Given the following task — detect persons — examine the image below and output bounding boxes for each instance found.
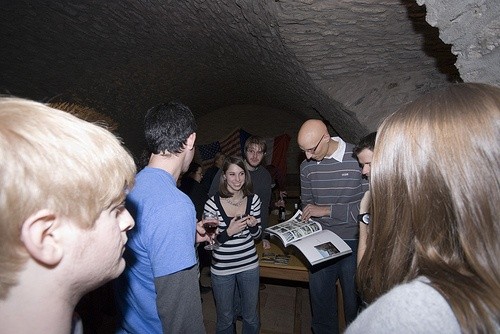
[202,156,262,334]
[0,96,137,334]
[180,119,377,334]
[341,83,500,334]
[115,103,219,333]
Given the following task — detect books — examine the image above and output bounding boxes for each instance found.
[263,209,352,266]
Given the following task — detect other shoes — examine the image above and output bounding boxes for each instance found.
[259,284,266,290]
[199,282,211,294]
[237,316,242,321]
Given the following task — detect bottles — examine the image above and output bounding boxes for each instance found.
[279,201,285,221]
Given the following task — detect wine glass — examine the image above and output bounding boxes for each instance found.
[202,211,219,250]
[235,214,247,238]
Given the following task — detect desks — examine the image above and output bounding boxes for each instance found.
[254,215,347,334]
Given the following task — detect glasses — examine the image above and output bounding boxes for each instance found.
[303,134,326,155]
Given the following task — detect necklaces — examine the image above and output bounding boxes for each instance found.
[224,196,246,207]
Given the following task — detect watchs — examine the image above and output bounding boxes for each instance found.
[358,212,370,225]
